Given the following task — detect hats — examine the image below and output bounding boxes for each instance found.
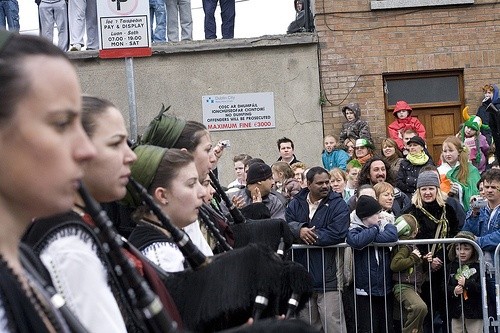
[407,136,425,147]
[247,162,273,183]
[447,231,479,263]
[141,103,186,147]
[355,196,382,219]
[417,171,440,188]
[351,139,375,149]
[461,106,490,164]
[394,217,411,237]
[116,144,167,210]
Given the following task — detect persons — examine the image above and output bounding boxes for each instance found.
[165,0,193,41]
[202,0,236,40]
[99,103,316,329]
[387,100,427,151]
[201,107,500,333]
[0,0,21,33]
[34,0,98,51]
[19,94,321,333]
[476,84,500,145]
[0,29,96,333]
[149,0,167,41]
[339,103,373,146]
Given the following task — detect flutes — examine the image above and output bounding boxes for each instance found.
[78,170,314,333]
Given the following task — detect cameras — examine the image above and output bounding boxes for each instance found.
[471,196,486,207]
[219,140,230,147]
